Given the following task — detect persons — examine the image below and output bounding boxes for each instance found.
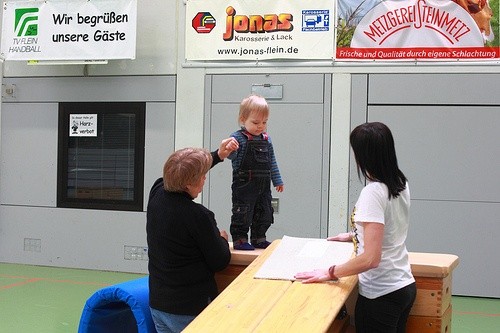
[226,96,284,250]
[145,137,239,333]
[295,122,417,333]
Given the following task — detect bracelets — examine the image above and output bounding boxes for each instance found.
[329,265,337,279]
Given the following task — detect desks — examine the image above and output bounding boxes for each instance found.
[217,243,460,333]
[180,236,356,333]
